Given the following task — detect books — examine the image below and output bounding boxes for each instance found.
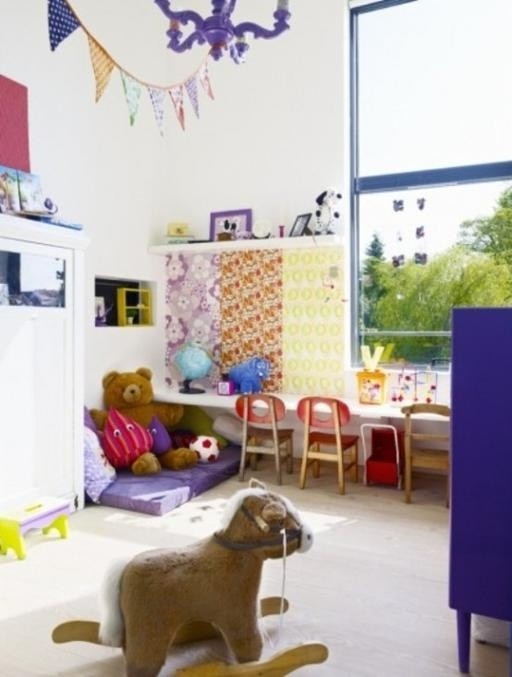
[17,169,51,214]
[37,198,84,230]
[0,164,21,215]
[19,207,48,217]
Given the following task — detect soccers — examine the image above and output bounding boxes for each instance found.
[189,435,219,463]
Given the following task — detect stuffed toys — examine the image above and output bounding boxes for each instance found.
[228,357,270,395]
[88,367,198,476]
[314,188,342,235]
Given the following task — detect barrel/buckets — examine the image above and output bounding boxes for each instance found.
[356,368,388,405]
